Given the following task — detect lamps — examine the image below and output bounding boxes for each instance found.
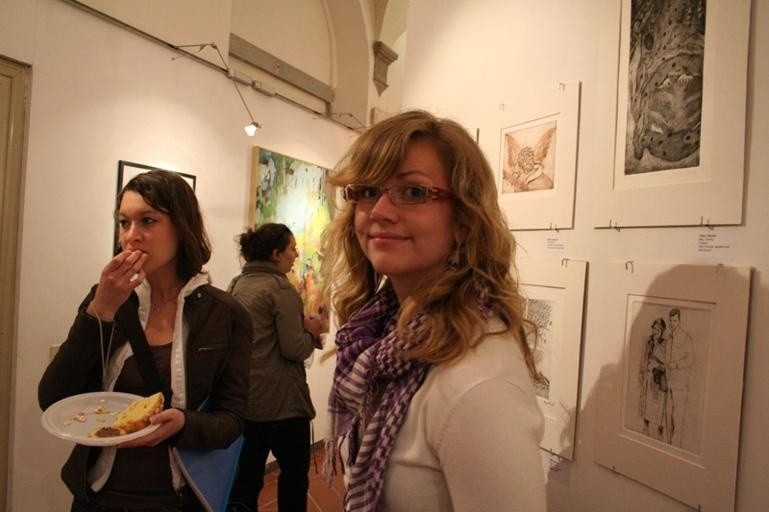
[166,41,264,140]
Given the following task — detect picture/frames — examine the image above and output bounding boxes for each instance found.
[482,79,585,233]
[508,255,589,465]
[112,159,198,257]
[589,0,756,232]
[591,255,759,512]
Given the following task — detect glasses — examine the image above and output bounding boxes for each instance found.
[341,181,450,205]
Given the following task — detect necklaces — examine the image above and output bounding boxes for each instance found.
[149,286,180,313]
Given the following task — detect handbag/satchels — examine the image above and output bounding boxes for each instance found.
[179,448,264,511]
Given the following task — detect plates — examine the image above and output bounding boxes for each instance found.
[41,390,161,450]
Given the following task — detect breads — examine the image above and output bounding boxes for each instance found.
[93,390,164,439]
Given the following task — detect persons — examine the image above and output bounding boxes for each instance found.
[318,107,550,511]
[36,168,254,511]
[637,317,672,442]
[226,222,321,512]
[665,306,694,448]
